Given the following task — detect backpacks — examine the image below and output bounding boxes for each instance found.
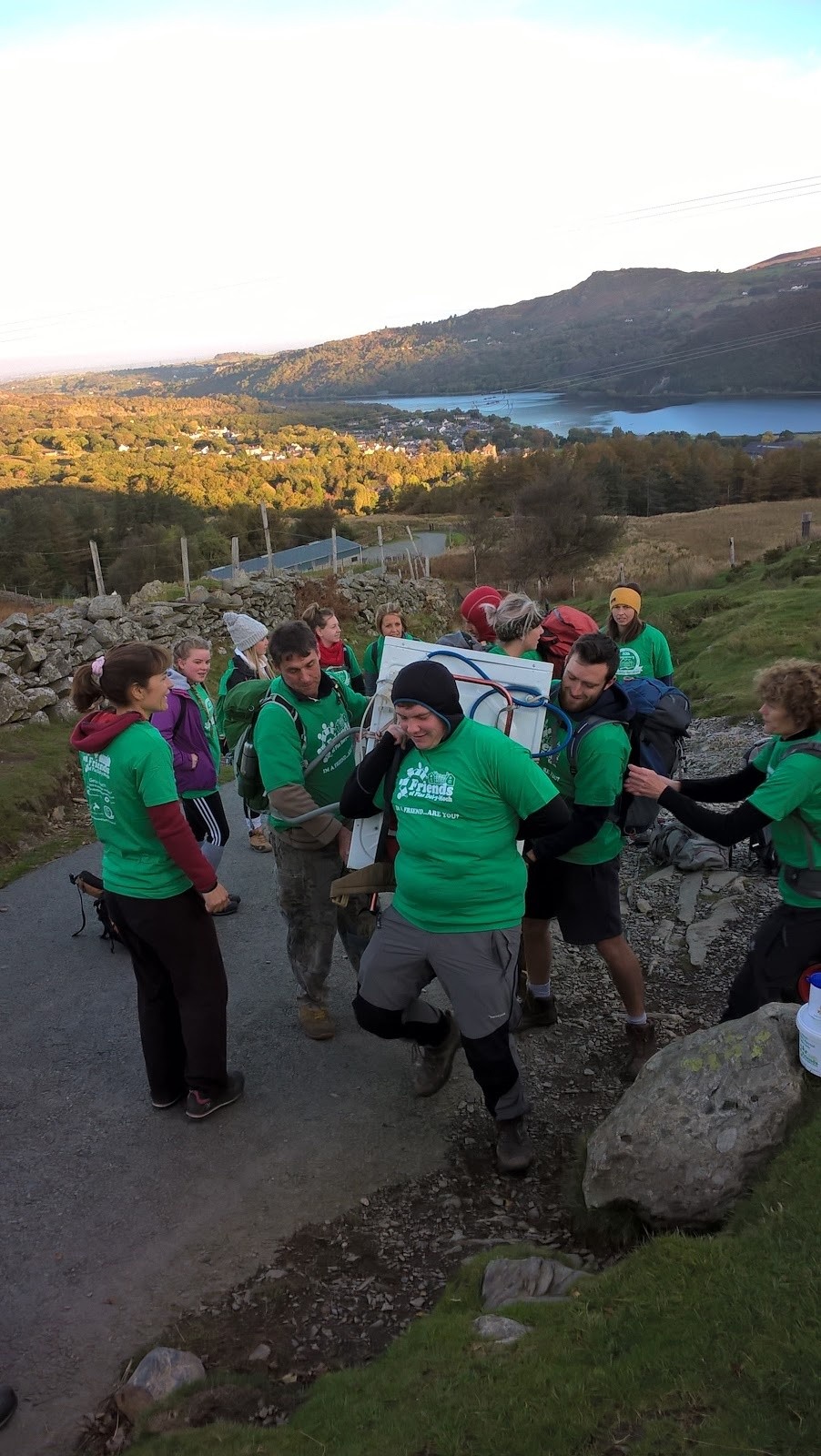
[537,605,599,681]
[650,821,728,873]
[221,679,269,811]
[568,676,692,835]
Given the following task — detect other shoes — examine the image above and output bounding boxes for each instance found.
[186,1070,246,1119]
[152,1094,179,1107]
[212,895,241,916]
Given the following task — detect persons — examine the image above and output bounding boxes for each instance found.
[66,643,247,1118]
[621,658,821,1023]
[214,583,673,853]
[339,658,572,1173]
[509,631,658,1081]
[150,635,241,916]
[252,622,382,1039]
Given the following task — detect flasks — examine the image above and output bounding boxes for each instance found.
[240,739,258,780]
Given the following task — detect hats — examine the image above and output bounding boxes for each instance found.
[223,612,268,652]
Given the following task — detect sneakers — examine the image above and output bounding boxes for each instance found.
[624,1019,656,1080]
[411,1009,464,1097]
[517,988,558,1032]
[248,827,272,852]
[495,1121,531,1179]
[296,984,334,1040]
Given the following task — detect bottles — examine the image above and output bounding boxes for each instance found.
[795,972,821,1077]
